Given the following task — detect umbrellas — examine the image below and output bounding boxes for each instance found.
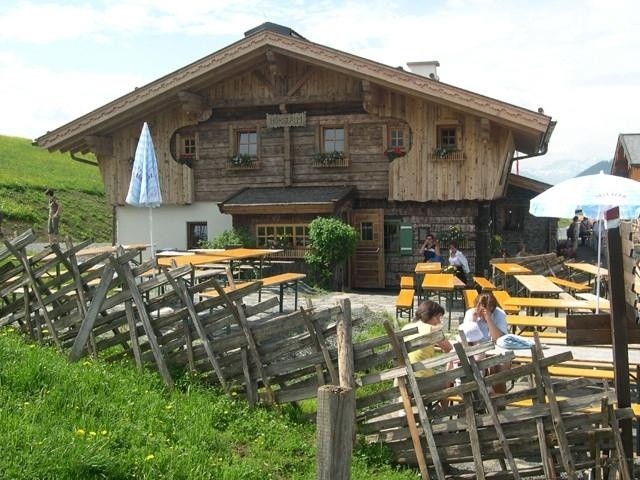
[529,169,640,314]
[125,120,163,278]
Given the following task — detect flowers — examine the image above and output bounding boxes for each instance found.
[173,144,456,169]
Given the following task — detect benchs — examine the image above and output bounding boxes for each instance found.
[139,245,307,318]
[385,261,640,421]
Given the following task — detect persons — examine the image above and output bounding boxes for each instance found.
[447,240,471,285]
[44,189,61,248]
[393,300,454,417]
[420,233,446,265]
[565,215,592,255]
[458,293,514,410]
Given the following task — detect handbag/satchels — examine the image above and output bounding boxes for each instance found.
[495,333,533,351]
